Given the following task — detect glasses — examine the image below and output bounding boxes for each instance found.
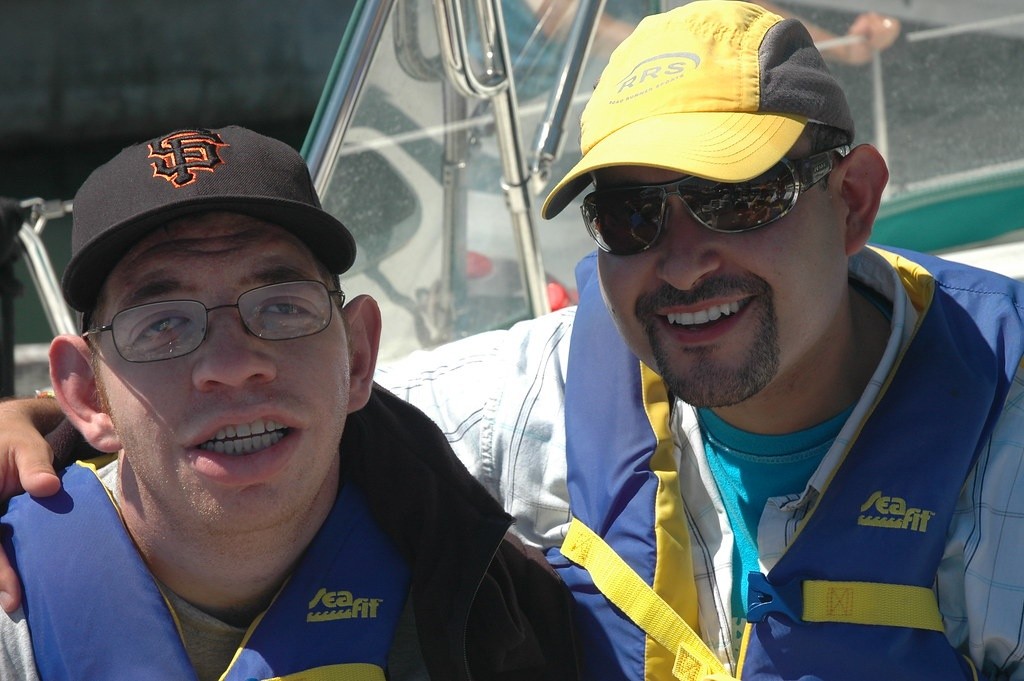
[580,139,848,257]
[84,278,348,365]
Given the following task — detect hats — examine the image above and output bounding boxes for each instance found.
[541,2,854,221]
[62,124,357,314]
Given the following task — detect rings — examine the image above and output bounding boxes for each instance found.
[882,19,892,30]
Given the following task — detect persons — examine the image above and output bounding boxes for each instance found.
[496,1,902,319]
[4,0,1024,680]
[0,123,622,681]
